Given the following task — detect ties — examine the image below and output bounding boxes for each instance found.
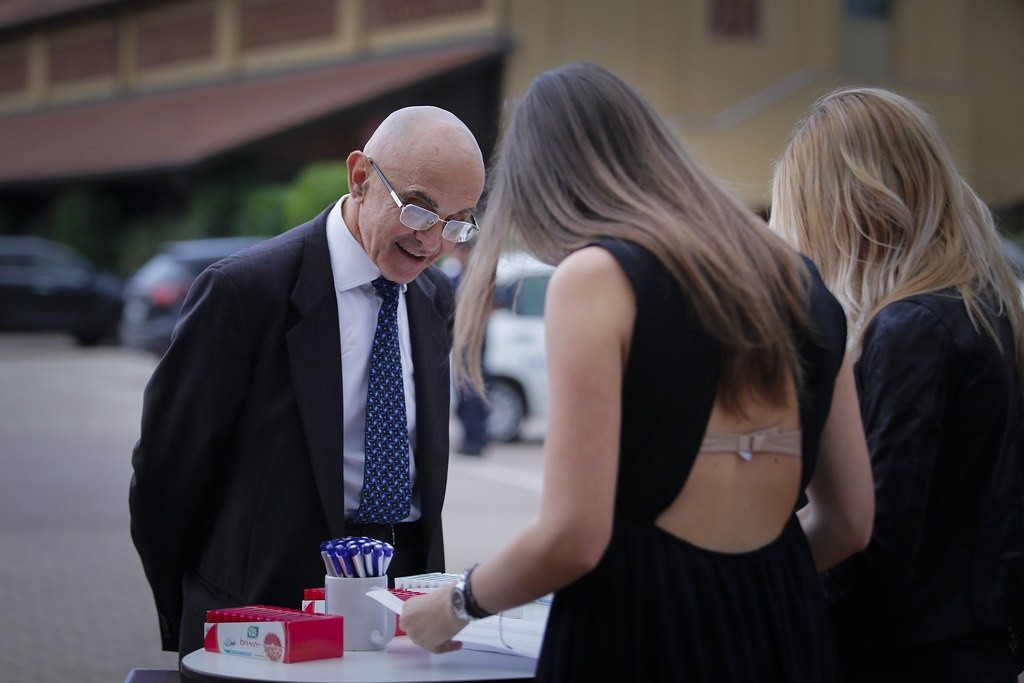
[353,275,413,524]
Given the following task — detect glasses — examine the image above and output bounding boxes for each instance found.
[366,156,480,243]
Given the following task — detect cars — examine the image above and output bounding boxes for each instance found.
[0,232,130,348]
[119,235,277,357]
[432,248,560,450]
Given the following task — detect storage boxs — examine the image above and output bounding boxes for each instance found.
[302,599,406,637]
[203,615,344,663]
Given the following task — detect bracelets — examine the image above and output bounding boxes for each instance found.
[463,565,492,619]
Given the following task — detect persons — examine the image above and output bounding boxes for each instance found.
[399,62,877,683]
[766,85,1023,683]
[128,106,486,683]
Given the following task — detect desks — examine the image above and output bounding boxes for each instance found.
[179,632,538,683]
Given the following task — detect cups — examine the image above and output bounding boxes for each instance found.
[325,574,397,651]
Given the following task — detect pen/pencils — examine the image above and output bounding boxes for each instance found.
[320,536,393,578]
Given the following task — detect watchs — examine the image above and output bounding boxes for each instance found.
[451,564,477,621]
